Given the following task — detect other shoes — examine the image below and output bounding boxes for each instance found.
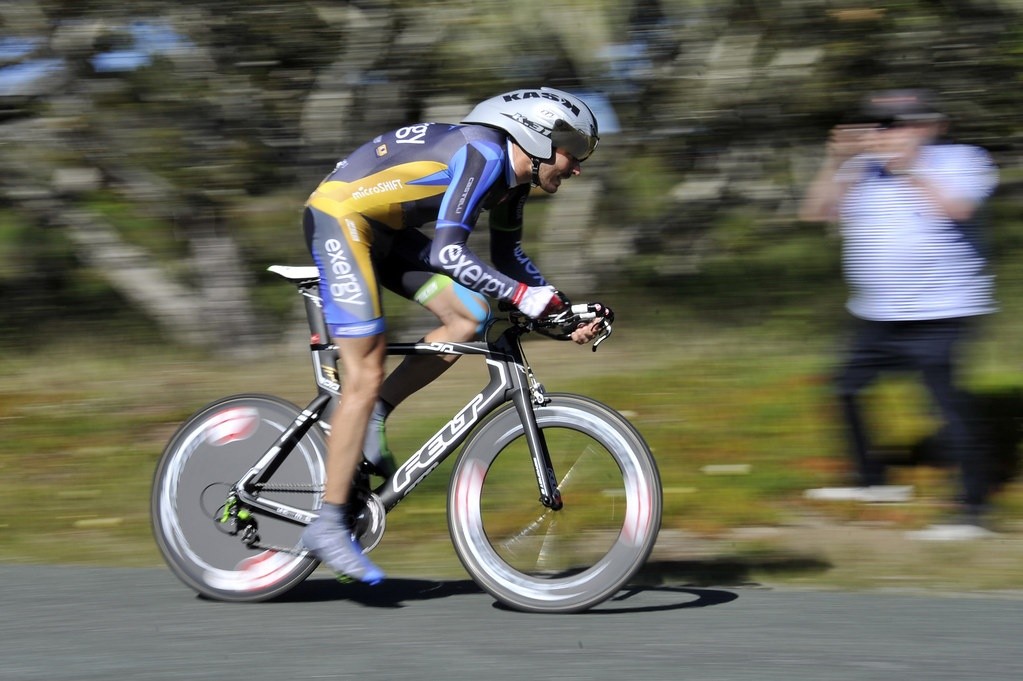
[360,404,397,479]
[303,502,384,585]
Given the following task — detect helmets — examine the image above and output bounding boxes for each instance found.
[459,86,600,162]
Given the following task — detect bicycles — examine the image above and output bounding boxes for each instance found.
[150,265,662,614]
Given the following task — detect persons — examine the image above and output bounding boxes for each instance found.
[795,84,1004,540]
[301,86,604,586]
